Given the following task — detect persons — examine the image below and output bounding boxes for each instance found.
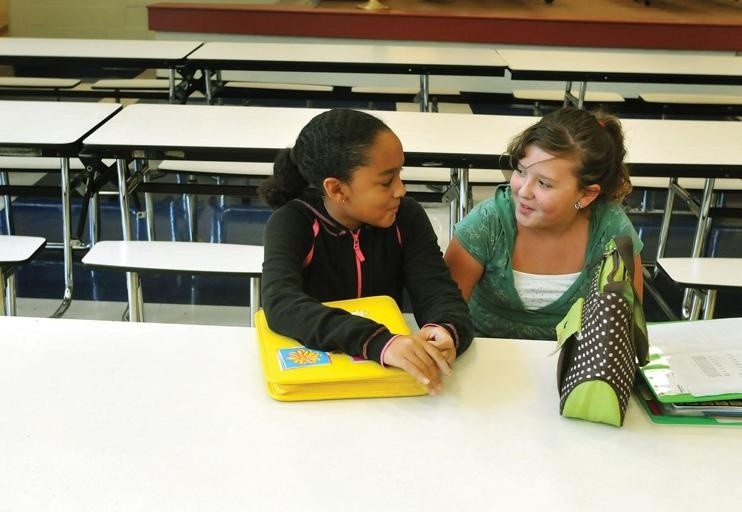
[254,105,477,399]
[442,104,647,341]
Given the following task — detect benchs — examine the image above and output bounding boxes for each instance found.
[0,37,742,328]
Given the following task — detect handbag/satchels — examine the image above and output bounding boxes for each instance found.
[551,234,650,428]
[255,295,431,402]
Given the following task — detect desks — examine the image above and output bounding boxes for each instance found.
[0,315,742,440]
